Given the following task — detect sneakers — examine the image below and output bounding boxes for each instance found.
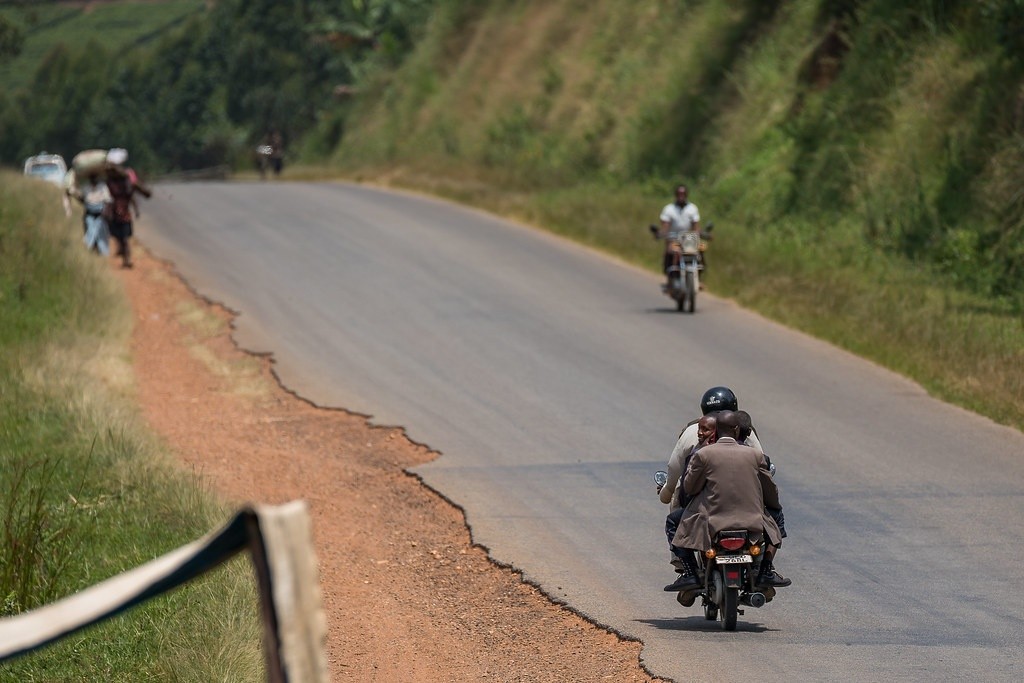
[663,574,700,591]
[755,570,792,587]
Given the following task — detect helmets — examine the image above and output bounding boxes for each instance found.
[701,387,739,416]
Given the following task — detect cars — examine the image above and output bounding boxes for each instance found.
[24,154,69,190]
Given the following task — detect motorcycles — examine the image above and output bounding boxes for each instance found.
[652,464,776,633]
[649,220,717,316]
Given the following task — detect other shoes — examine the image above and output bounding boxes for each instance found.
[756,586,775,602]
[676,591,695,607]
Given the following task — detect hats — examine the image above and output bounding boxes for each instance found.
[107,147,128,165]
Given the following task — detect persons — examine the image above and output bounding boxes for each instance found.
[658,387,791,593]
[253,129,286,179]
[652,185,713,288]
[67,157,153,270]
